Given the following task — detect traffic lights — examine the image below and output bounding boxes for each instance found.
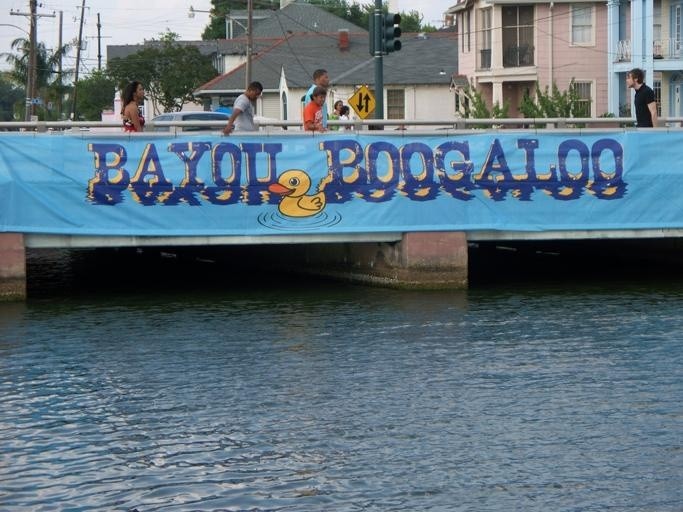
[379,11,401,54]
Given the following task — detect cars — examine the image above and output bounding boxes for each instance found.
[143,111,236,132]
[47,117,92,131]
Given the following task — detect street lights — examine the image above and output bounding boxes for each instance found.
[187,0,252,97]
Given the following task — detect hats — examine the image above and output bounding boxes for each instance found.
[310,87,326,100]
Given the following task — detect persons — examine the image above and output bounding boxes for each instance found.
[332,100,349,131]
[224,82,263,136]
[626,69,658,128]
[122,81,144,132]
[395,125,407,130]
[304,69,329,131]
